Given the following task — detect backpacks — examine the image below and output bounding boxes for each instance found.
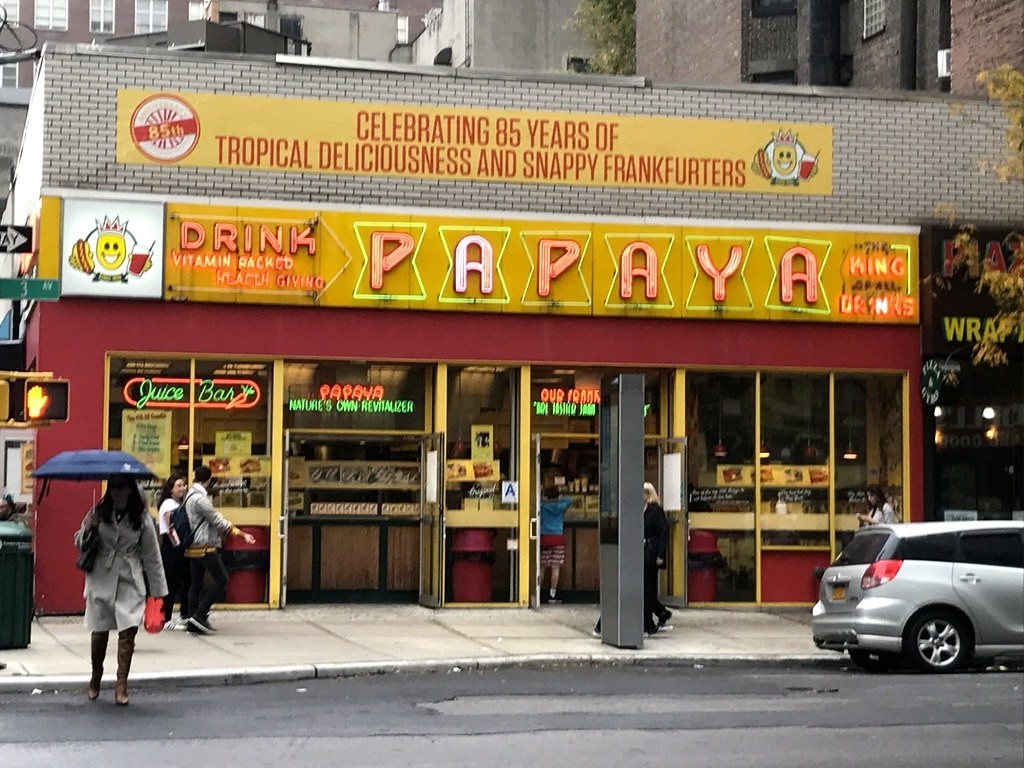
[164,493,208,549]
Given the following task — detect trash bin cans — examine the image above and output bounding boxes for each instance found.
[226,526,267,602]
[687,530,720,602]
[450,529,494,604]
[0,521,33,649]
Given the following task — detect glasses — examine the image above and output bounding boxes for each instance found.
[108,480,128,491]
[174,485,187,490]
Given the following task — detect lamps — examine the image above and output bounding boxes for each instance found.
[843,375,858,460]
[760,380,770,459]
[804,373,817,457]
[714,373,727,456]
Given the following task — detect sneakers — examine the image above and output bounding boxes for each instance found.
[163,619,187,630]
[186,623,202,635]
[189,612,217,634]
[177,616,189,625]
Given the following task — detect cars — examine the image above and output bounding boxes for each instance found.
[810,520,1024,672]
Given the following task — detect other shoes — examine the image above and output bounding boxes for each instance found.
[547,593,562,604]
[592,630,601,639]
[649,626,673,637]
[657,611,671,625]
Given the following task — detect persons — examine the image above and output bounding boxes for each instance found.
[157,473,187,631]
[591,591,674,638]
[539,486,572,604]
[73,475,169,706]
[643,481,672,633]
[29,448,160,513]
[178,466,256,635]
[856,483,896,527]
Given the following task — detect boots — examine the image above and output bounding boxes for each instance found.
[88,637,106,699]
[116,638,134,704]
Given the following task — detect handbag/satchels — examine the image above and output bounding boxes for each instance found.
[75,505,100,572]
[145,595,165,633]
